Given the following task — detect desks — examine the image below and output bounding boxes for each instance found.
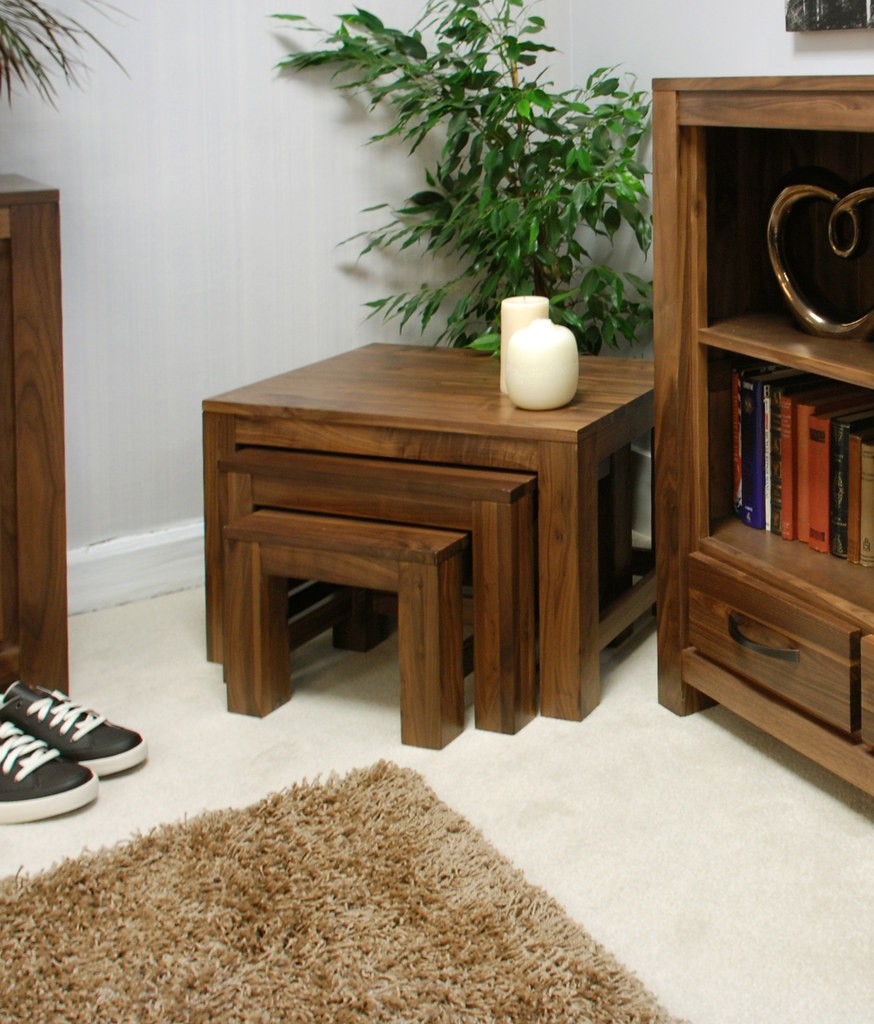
[652,76,874,796]
[0,173,69,699]
[202,341,656,723]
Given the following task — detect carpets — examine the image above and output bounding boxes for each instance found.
[0,757,698,1024]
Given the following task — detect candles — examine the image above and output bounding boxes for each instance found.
[500,295,549,394]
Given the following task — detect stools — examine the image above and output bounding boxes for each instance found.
[216,445,634,735]
[221,510,471,752]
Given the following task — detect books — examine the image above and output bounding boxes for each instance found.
[730,365,874,572]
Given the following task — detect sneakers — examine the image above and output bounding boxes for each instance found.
[0,678,147,777]
[0,718,102,825]
